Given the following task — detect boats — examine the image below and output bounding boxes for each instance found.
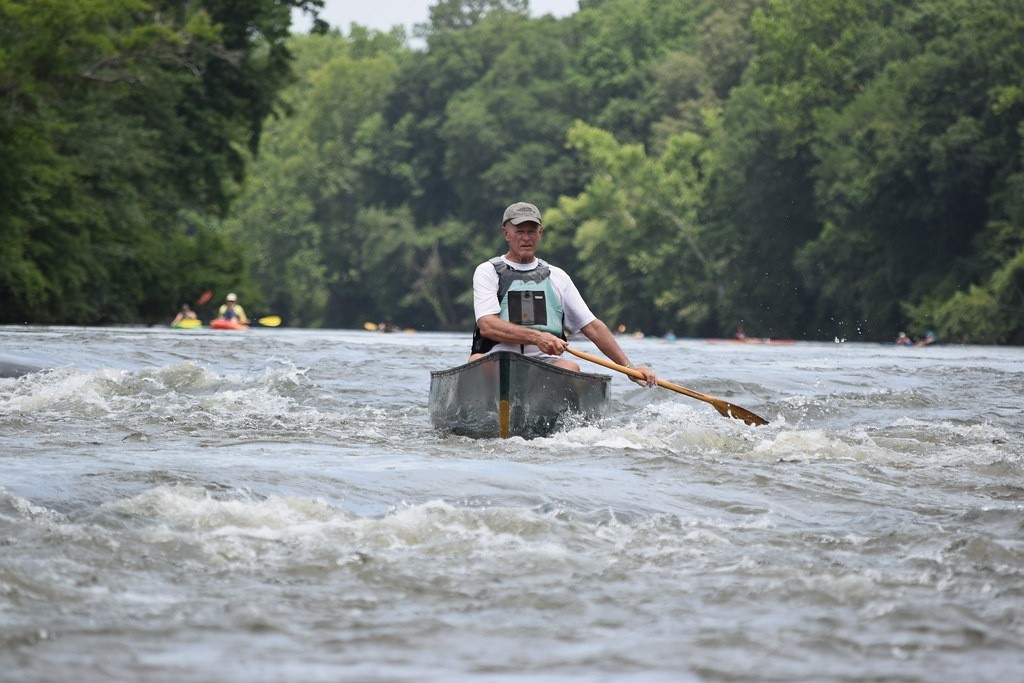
[210,318,246,330]
[708,338,798,345]
[175,319,201,329]
[898,332,937,347]
[428,350,612,439]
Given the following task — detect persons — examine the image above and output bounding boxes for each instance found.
[736,326,747,339]
[468,202,658,388]
[218,293,247,325]
[175,305,197,320]
[896,332,912,345]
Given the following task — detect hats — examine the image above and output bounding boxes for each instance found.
[227,294,236,301]
[502,202,542,225]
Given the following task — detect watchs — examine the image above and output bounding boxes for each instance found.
[625,361,634,367]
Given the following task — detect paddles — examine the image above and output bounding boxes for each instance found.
[177,315,282,329]
[562,344,772,429]
[364,321,415,334]
[180,290,213,321]
